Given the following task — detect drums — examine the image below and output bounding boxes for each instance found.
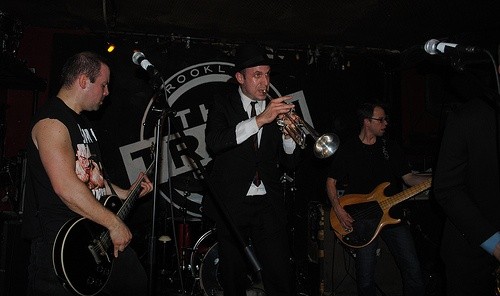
[166,217,202,251]
[190,228,256,296]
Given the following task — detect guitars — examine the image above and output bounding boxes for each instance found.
[329,178,433,249]
[52,140,160,296]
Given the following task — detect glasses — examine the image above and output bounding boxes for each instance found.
[365,114,390,124]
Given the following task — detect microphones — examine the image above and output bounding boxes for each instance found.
[131,51,160,77]
[423,39,480,55]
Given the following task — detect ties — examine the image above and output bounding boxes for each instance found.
[250,100,261,187]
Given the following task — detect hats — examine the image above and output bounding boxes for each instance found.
[225,44,276,75]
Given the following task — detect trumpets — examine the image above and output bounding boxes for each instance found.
[263,89,339,160]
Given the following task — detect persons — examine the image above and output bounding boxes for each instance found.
[428,58,500,296]
[326,103,431,296]
[26,51,153,296]
[204,46,300,296]
